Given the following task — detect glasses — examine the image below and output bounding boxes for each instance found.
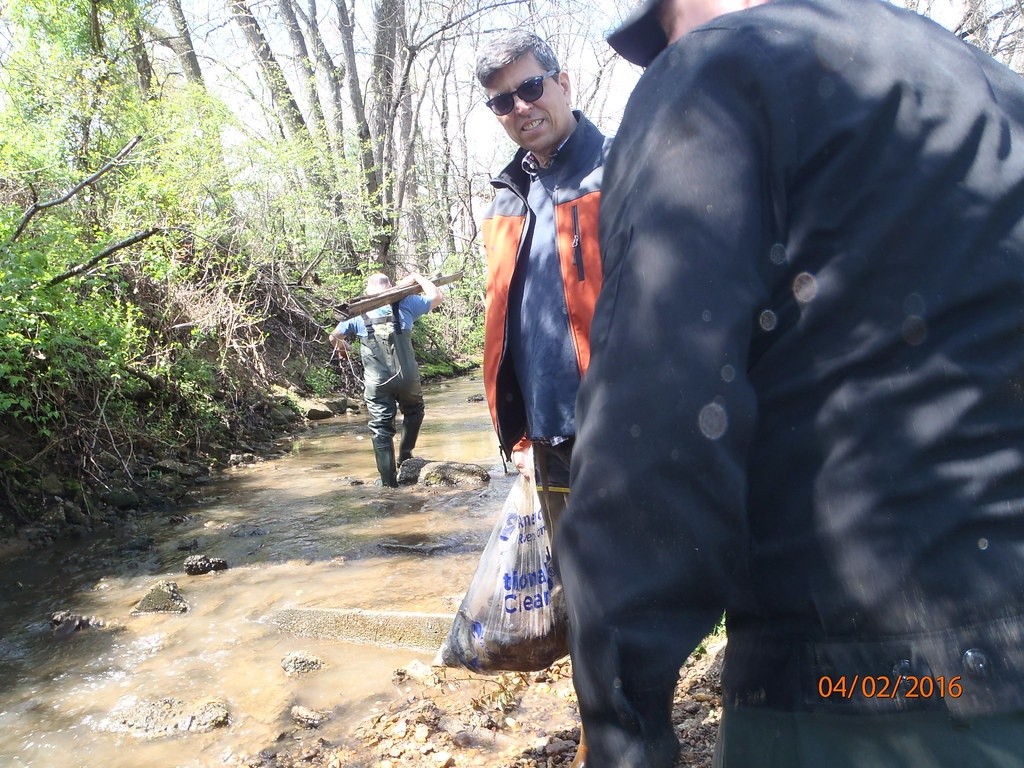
[485,68,557,116]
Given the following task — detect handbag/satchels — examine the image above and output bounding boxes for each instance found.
[441,451,572,673]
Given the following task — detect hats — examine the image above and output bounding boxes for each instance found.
[363,274,393,295]
[606,0,667,66]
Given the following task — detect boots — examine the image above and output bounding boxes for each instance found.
[373,436,397,489]
[396,412,423,469]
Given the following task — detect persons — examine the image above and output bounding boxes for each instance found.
[330,272,444,486]
[474,30,615,768]
[554,0,1024,768]
